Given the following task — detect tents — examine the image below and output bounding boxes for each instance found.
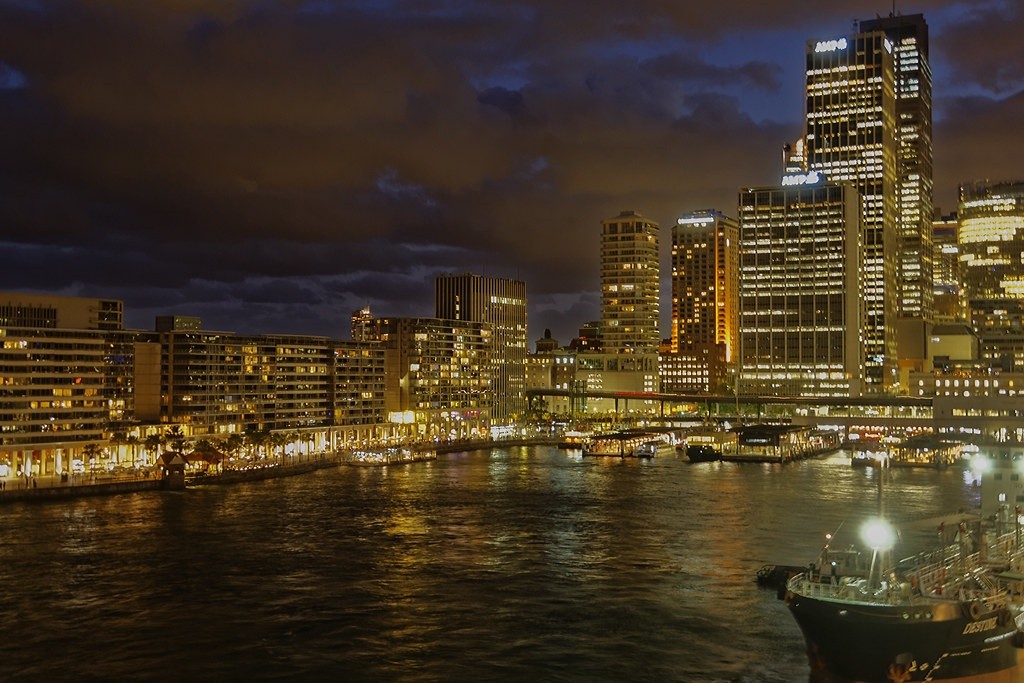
[184,447,230,469]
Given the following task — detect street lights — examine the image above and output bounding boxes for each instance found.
[855,449,995,581]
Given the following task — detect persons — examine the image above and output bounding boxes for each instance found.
[33,478,36,488]
[0,480,6,490]
